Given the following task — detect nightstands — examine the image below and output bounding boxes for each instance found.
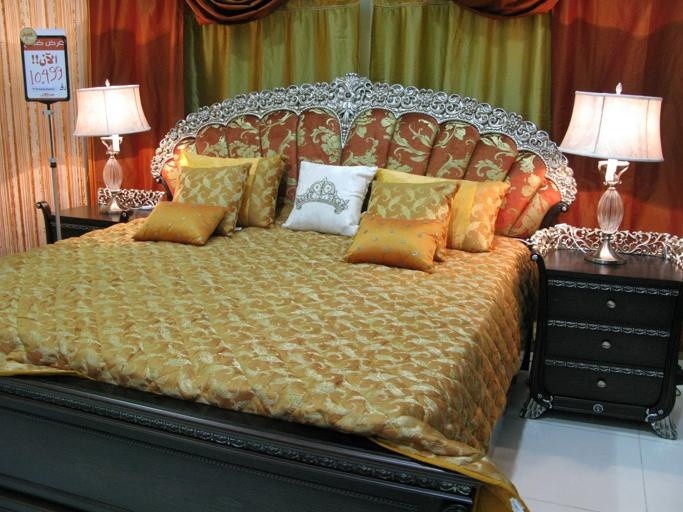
[518,247,682,441]
[34,201,151,245]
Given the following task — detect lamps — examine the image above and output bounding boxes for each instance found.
[71,77,151,215]
[555,83,664,266]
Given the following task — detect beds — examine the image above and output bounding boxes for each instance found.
[0,73,578,510]
